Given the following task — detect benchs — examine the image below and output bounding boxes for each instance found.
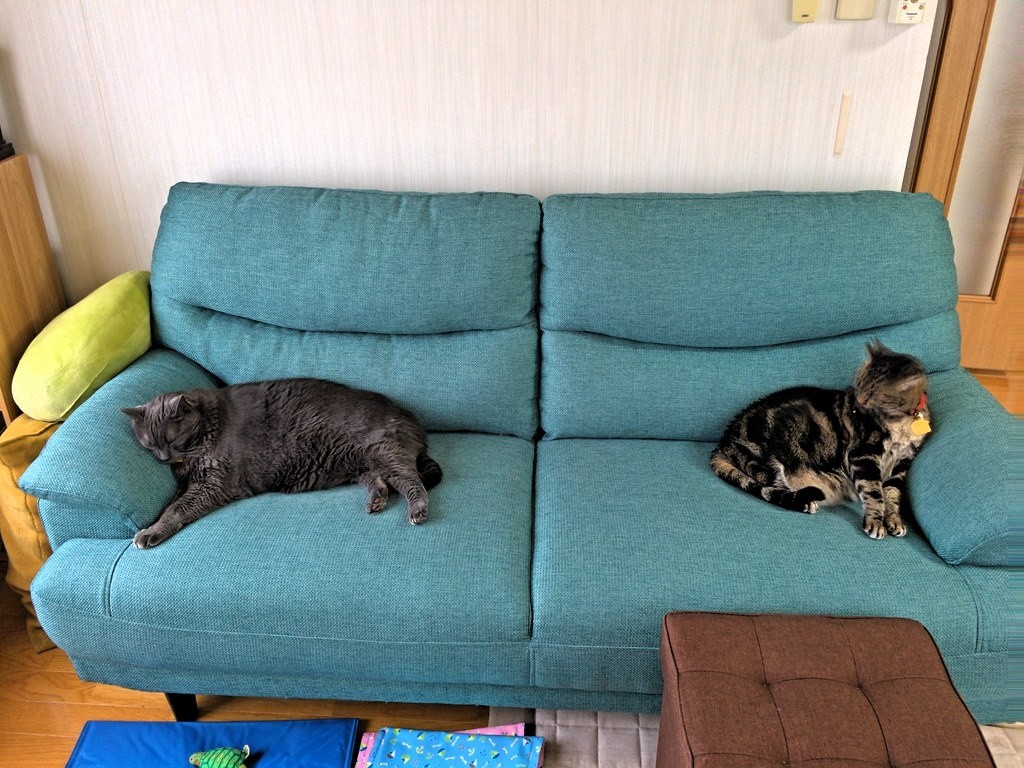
[654,613,999,768]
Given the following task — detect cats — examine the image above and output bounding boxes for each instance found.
[709,334,933,540]
[120,376,444,552]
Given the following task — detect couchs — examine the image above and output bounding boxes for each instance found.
[15,181,1024,722]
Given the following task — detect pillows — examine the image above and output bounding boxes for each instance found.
[12,269,152,421]
[0,413,62,655]
